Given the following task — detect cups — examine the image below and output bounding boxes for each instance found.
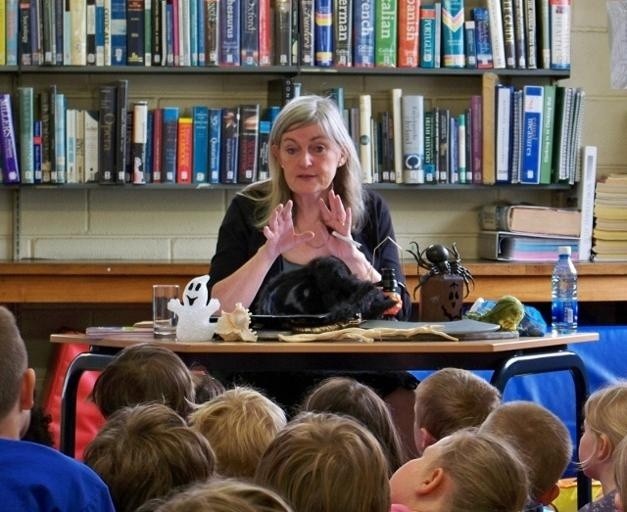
[417,273,463,323]
[151,283,178,338]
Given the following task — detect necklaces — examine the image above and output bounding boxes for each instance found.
[294,215,332,250]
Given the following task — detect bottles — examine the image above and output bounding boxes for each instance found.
[550,245,579,335]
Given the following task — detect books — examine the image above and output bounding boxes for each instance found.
[477,169,626,262]
[0,1,572,70]
[0,73,586,185]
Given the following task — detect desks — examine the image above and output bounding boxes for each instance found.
[52,328,600,512]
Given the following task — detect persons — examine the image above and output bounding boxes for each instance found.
[203,93,412,320]
[81,343,624,512]
[0,306,115,512]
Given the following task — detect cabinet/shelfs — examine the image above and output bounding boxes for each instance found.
[0,0,575,191]
